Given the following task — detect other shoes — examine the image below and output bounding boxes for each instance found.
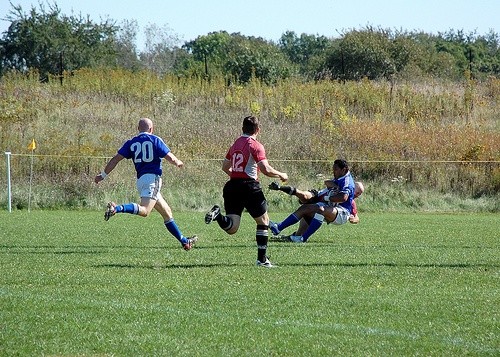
[281,231,297,242]
[205,205,221,224]
[289,235,309,243]
[269,220,281,236]
[257,255,279,268]
[269,182,280,190]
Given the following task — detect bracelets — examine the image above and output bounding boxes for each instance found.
[100,171,108,179]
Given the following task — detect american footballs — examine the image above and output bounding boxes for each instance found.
[327,187,341,207]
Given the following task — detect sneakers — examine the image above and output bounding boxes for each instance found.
[182,235,198,251]
[104,201,118,221]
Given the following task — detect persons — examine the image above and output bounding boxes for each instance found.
[95,118,198,251]
[268,159,355,243]
[269,181,364,240]
[204,116,288,268]
